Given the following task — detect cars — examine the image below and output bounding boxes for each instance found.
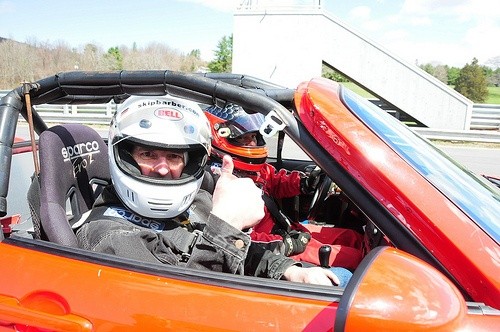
[0,68,499,332]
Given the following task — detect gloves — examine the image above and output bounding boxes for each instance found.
[282,230,311,257]
[300,169,326,194]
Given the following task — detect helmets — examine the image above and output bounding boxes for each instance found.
[108,92,210,218]
[203,103,269,175]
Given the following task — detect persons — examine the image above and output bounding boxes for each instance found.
[75,92,354,288]
[204,101,366,270]
